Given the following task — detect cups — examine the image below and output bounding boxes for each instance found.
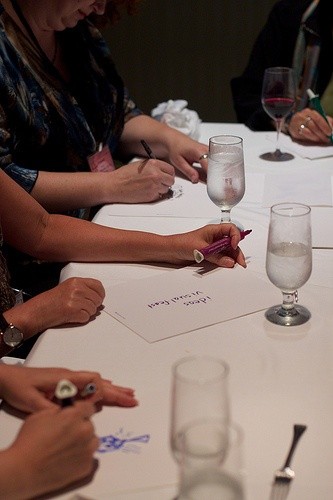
[170,358,230,469]
[167,420,247,500]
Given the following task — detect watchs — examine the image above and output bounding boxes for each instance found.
[0,312,25,349]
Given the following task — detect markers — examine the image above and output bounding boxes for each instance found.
[193,230,252,263]
[55,379,78,406]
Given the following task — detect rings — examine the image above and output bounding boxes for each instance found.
[297,124,306,135]
[305,117,311,125]
[199,154,207,160]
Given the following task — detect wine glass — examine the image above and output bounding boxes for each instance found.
[263,203,313,327]
[260,67,298,162]
[206,134,246,232]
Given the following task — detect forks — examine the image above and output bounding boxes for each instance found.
[269,422,308,500]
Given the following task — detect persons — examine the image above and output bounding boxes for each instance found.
[0,0,220,301]
[0,362,139,500]
[228,0,333,143]
[0,167,246,351]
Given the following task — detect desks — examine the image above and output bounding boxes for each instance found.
[0,123,333,500]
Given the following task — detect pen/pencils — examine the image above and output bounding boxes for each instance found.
[306,88,333,144]
[81,383,96,397]
[141,140,156,159]
